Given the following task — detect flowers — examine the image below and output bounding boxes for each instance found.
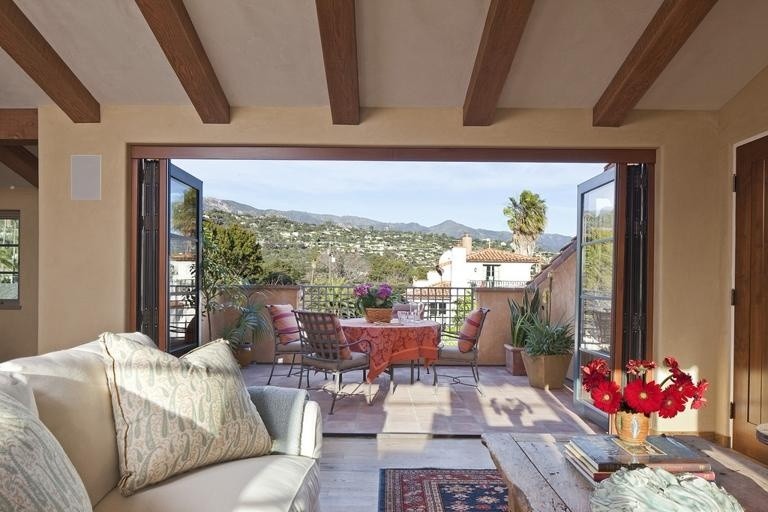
[580,357,709,418]
[351,283,400,315]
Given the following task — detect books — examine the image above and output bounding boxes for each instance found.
[563,433,717,488]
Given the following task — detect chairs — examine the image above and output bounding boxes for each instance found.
[265,304,327,388]
[388,302,430,382]
[292,309,373,414]
[433,306,490,387]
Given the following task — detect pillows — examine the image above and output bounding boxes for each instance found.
[97,332,273,497]
[458,307,483,352]
[269,304,301,344]
[298,311,353,359]
[0,368,96,512]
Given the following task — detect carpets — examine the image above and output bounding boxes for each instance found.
[378,468,513,512]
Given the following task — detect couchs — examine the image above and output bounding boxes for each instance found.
[0,331,323,512]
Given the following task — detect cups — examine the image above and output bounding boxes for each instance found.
[396,311,416,325]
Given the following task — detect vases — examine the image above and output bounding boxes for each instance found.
[615,410,650,445]
[364,308,393,323]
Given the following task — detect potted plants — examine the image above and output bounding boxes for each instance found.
[219,284,278,367]
[504,286,541,375]
[519,308,575,390]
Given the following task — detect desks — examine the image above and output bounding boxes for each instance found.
[477,433,768,512]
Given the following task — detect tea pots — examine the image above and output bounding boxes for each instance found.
[409,302,424,320]
